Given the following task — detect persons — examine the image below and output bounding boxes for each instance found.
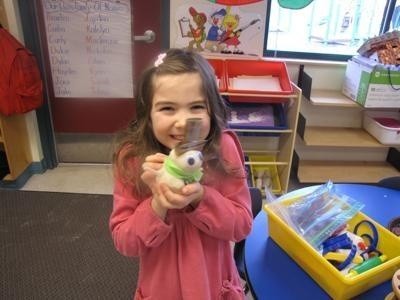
[105,48,255,300]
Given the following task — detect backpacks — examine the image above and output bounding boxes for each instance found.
[0,24,44,118]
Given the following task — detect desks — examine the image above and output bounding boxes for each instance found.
[243,181,400,300]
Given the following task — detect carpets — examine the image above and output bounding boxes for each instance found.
[0,188,139,300]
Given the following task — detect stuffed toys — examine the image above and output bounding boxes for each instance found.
[156,117,209,195]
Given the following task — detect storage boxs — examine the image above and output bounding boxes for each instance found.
[341,58,399,145]
[243,153,282,197]
[261,196,400,299]
[203,54,294,138]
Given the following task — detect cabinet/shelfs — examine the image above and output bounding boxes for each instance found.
[214,81,303,197]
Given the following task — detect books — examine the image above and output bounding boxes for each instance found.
[356,30,400,56]
